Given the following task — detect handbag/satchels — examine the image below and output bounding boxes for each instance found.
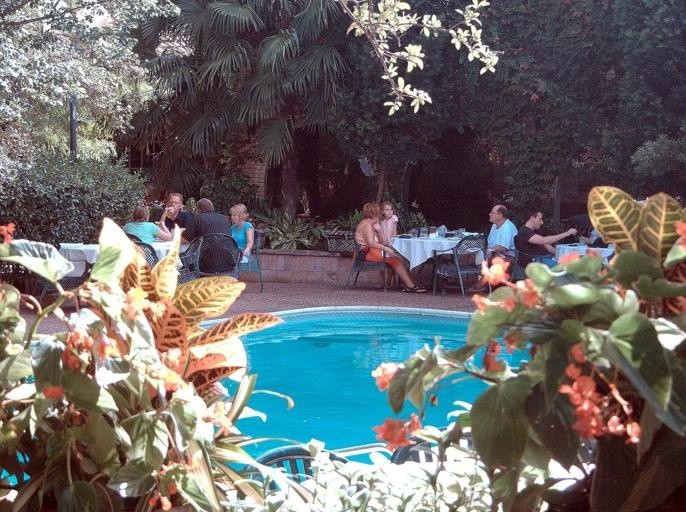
[356,250,366,261]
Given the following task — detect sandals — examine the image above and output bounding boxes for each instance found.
[406,285,428,294]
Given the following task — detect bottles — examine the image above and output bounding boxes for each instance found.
[438,224,448,236]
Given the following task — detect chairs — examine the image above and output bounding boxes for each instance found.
[344,228,618,298]
[1,232,100,304]
[391,426,475,464]
[236,445,349,500]
[127,230,263,293]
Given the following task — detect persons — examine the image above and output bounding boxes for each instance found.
[468,204,518,290]
[518,208,578,269]
[583,237,608,248]
[355,202,428,293]
[379,202,399,289]
[122,192,255,277]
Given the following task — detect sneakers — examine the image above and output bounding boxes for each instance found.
[464,286,488,294]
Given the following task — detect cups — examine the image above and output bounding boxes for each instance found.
[169,209,174,215]
[430,227,436,239]
[579,237,585,246]
[421,227,428,239]
[459,228,465,238]
